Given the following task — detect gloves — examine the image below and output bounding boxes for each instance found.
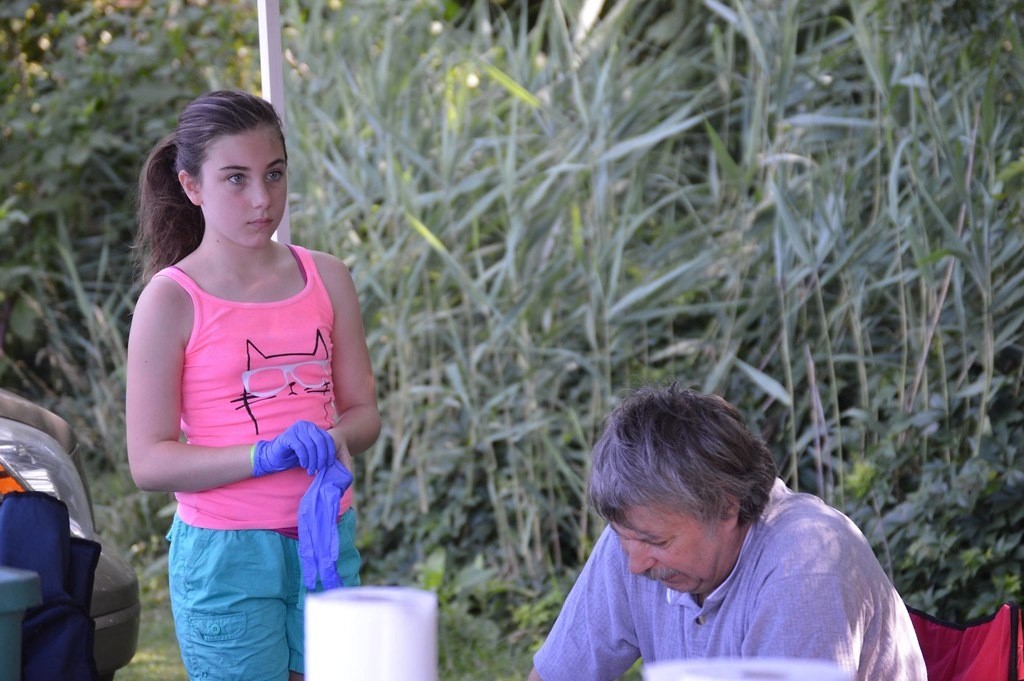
[298,459,354,591]
[253,419,335,476]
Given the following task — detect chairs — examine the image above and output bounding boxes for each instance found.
[906,599,1024,681]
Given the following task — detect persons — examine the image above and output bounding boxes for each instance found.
[125,90,381,681]
[527,380,932,681]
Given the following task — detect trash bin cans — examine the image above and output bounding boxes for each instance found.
[0,568,46,681]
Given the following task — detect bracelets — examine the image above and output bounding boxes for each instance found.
[250,443,259,470]
